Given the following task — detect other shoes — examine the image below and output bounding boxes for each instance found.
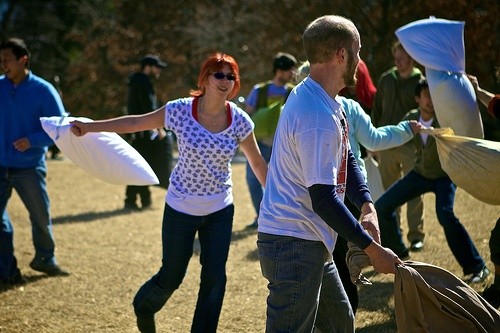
[142,205,160,210]
[29,259,69,276]
[411,240,423,251]
[50,154,57,159]
[121,206,142,212]
[2,273,25,284]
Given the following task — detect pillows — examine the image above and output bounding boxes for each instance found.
[248,99,280,143]
[394,15,485,139]
[38,116,161,194]
[363,156,385,204]
[422,119,500,207]
[147,132,174,190]
[345,227,500,333]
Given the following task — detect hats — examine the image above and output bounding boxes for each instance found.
[143,55,167,68]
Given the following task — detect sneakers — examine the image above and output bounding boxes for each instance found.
[246,220,258,229]
[466,266,490,283]
[132,301,157,333]
[395,249,409,260]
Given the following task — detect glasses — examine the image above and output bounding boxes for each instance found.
[206,73,236,81]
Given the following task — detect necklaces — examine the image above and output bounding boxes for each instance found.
[198,98,227,127]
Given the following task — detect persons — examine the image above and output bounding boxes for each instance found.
[466,68,500,308]
[257,15,404,333]
[69,52,268,333]
[298,60,423,316]
[374,78,491,284]
[48,76,63,159]
[371,42,427,251]
[0,37,70,285]
[337,58,378,160]
[125,54,167,211]
[245,52,298,229]
